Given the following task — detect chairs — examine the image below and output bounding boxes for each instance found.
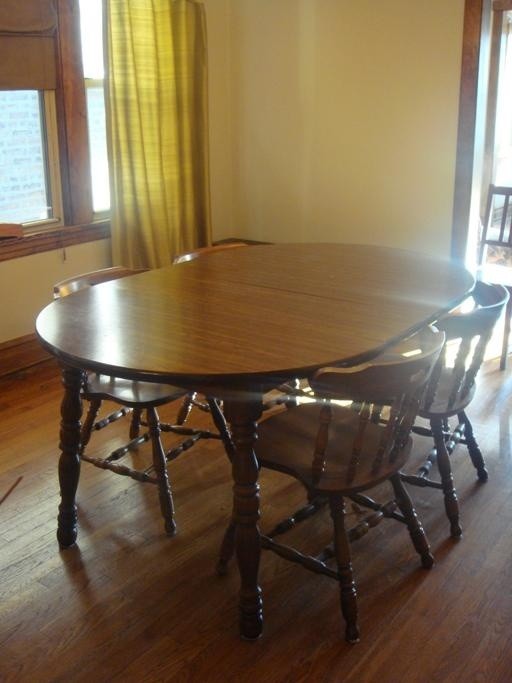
[52,266,233,538]
[475,183,512,372]
[348,283,509,544]
[171,243,300,426]
[217,328,447,642]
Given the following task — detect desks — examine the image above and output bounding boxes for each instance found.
[35,243,476,645]
